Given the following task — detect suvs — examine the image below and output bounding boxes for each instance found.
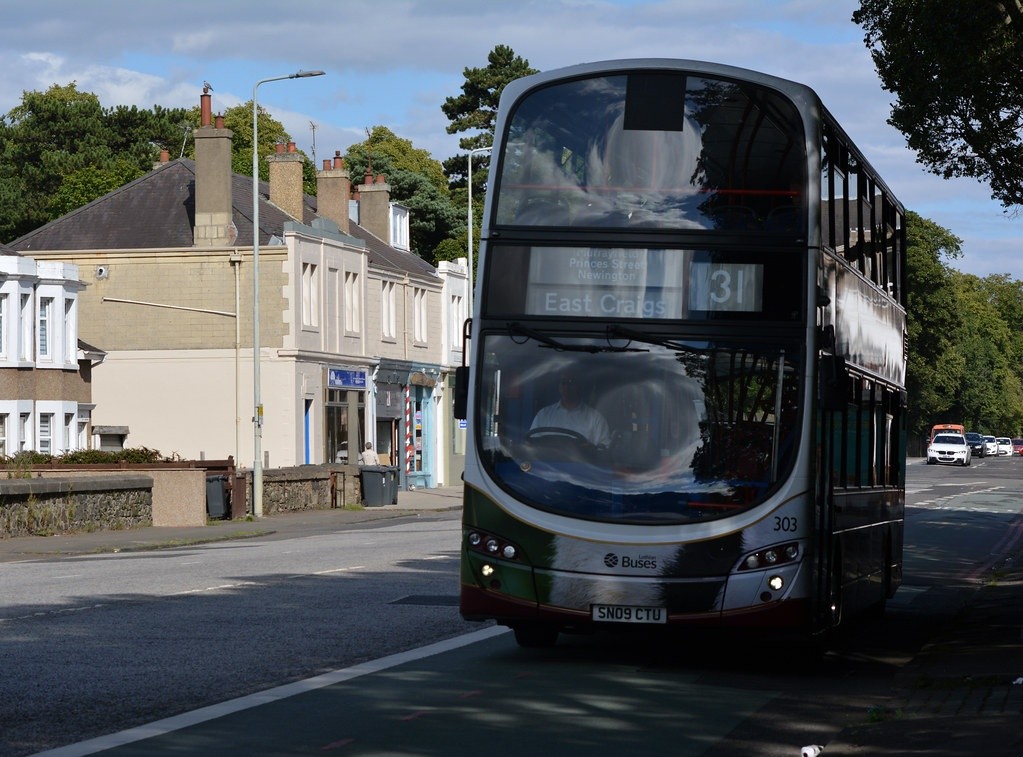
[965,433,987,459]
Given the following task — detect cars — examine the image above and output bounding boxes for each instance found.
[983,436,1023,457]
[926,433,972,466]
[335,441,348,464]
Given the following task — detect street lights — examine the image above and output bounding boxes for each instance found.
[468,141,525,363]
[252,69,326,518]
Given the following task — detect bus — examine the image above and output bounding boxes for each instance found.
[453,58,910,655]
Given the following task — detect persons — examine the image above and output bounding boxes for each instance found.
[362,442,379,465]
[529,364,610,449]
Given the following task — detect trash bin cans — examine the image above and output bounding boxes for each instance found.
[360,465,391,507]
[387,466,401,504]
[206,474,227,517]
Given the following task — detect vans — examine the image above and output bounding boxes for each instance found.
[929,424,964,447]
